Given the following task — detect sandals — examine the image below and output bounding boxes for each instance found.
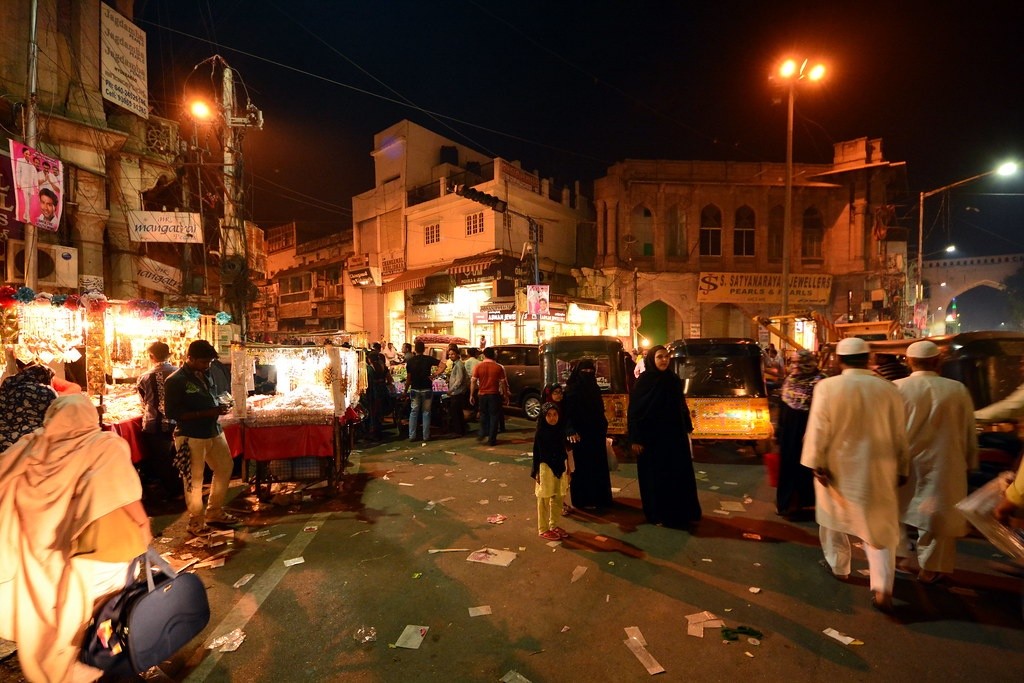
[186,513,214,537]
[204,508,238,524]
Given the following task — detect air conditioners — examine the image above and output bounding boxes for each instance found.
[4,238,78,289]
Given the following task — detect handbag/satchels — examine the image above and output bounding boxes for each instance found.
[79,546,210,676]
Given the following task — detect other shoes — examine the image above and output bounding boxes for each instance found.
[487,441,497,445]
[422,436,433,440]
[872,594,892,611]
[476,433,485,441]
[818,559,850,581]
[561,503,574,515]
[409,438,417,442]
[919,572,938,590]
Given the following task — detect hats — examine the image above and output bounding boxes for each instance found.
[836,338,871,355]
[905,340,941,358]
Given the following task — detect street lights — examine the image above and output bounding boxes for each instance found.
[190,101,212,305]
[915,161,1019,338]
[773,56,829,361]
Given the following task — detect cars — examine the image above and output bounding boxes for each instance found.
[480,343,543,422]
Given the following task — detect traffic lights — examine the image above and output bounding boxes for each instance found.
[492,200,507,213]
[454,184,498,207]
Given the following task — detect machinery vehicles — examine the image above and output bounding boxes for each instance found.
[752,310,908,372]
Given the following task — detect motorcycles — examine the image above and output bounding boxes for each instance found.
[542,335,638,445]
[666,337,773,452]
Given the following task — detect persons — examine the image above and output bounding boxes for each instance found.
[303,340,510,446]
[891,341,978,585]
[0,364,153,683]
[37,188,58,230]
[762,343,822,523]
[136,342,180,505]
[530,359,613,541]
[16,148,60,223]
[626,345,703,525]
[165,339,239,535]
[209,357,275,401]
[799,336,912,610]
[537,298,550,315]
[973,380,1024,522]
[480,335,486,348]
[631,347,648,379]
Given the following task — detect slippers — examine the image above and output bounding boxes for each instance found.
[539,530,560,540]
[552,527,568,538]
